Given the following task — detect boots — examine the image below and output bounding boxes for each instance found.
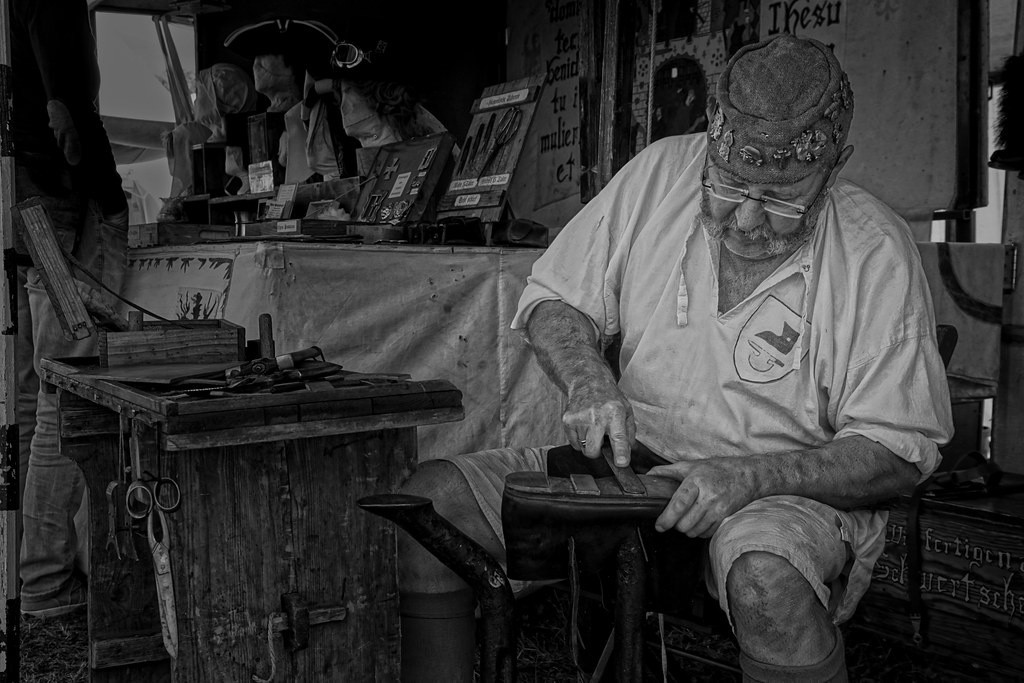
[399,588,480,683]
[738,624,848,683]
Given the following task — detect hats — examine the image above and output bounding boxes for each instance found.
[707,33,854,179]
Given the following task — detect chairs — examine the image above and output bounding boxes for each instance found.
[643,325,958,683]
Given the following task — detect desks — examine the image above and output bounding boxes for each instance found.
[38,356,466,683]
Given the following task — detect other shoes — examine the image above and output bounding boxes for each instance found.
[20,577,87,617]
[501,471,682,581]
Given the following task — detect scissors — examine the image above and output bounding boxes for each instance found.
[476,106,522,182]
[124,475,187,658]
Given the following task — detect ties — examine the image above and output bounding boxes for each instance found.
[700,151,837,219]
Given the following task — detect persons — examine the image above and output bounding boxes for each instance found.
[7,0,130,618]
[398,33,954,683]
